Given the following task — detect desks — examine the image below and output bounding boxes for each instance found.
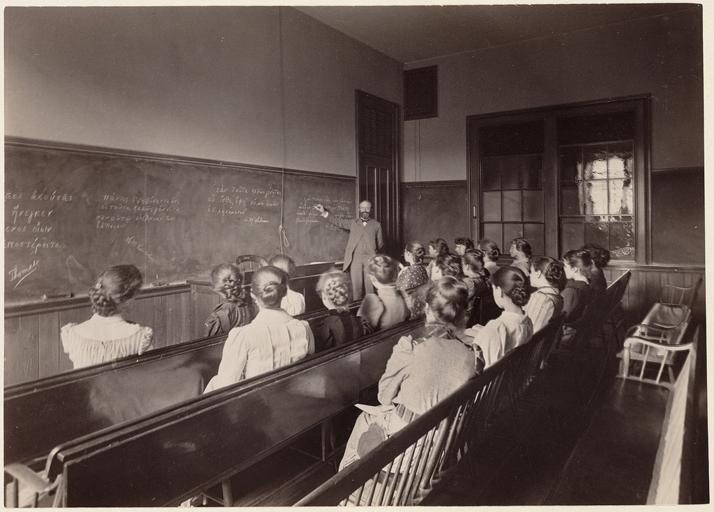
[186,262,336,339]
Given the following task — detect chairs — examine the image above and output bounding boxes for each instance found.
[555,267,633,349]
[1,343,362,504]
[363,315,433,408]
[289,308,571,506]
[535,340,705,504]
[7,295,376,506]
[614,272,705,390]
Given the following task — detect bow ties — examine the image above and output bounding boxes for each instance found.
[361,219,369,223]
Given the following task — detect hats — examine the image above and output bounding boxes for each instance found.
[395,265,429,291]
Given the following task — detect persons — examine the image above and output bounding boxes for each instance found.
[394,240,428,320]
[506,239,531,280]
[522,255,566,334]
[57,266,157,371]
[451,248,484,325]
[269,254,305,315]
[477,237,501,283]
[558,249,595,324]
[455,236,473,256]
[314,200,384,300]
[458,266,533,372]
[432,252,462,284]
[204,261,257,339]
[426,237,448,280]
[583,246,611,298]
[338,275,486,475]
[310,269,374,354]
[357,255,411,334]
[204,267,315,395]
